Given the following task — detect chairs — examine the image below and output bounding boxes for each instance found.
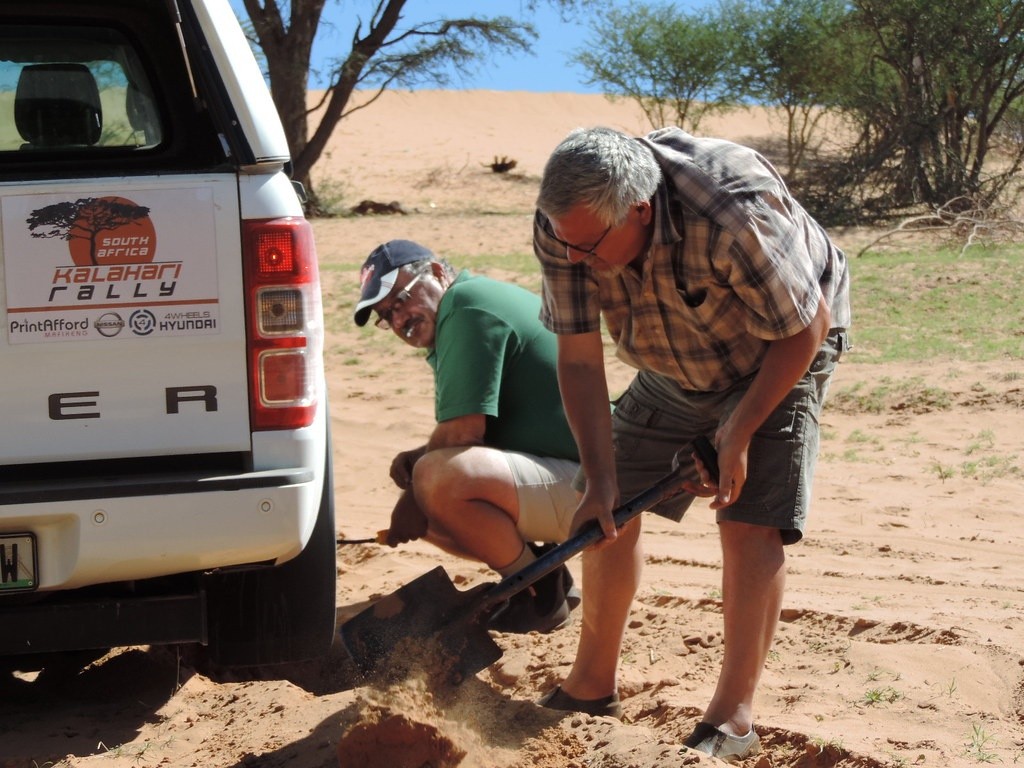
[7,61,105,153]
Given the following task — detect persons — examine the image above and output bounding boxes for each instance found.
[354,240,617,635]
[533,125,850,761]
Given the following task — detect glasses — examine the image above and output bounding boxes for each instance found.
[375,266,431,330]
[543,208,627,255]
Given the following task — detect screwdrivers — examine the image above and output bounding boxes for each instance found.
[338,530,391,548]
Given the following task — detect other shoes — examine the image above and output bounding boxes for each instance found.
[685,720,763,763]
[535,683,622,720]
[488,544,580,636]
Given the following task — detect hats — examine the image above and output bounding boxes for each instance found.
[354,240,433,326]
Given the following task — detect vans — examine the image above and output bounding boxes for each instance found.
[0,0,334,687]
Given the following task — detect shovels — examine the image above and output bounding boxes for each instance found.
[337,433,732,689]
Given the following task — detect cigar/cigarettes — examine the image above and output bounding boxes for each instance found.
[407,327,415,336]
